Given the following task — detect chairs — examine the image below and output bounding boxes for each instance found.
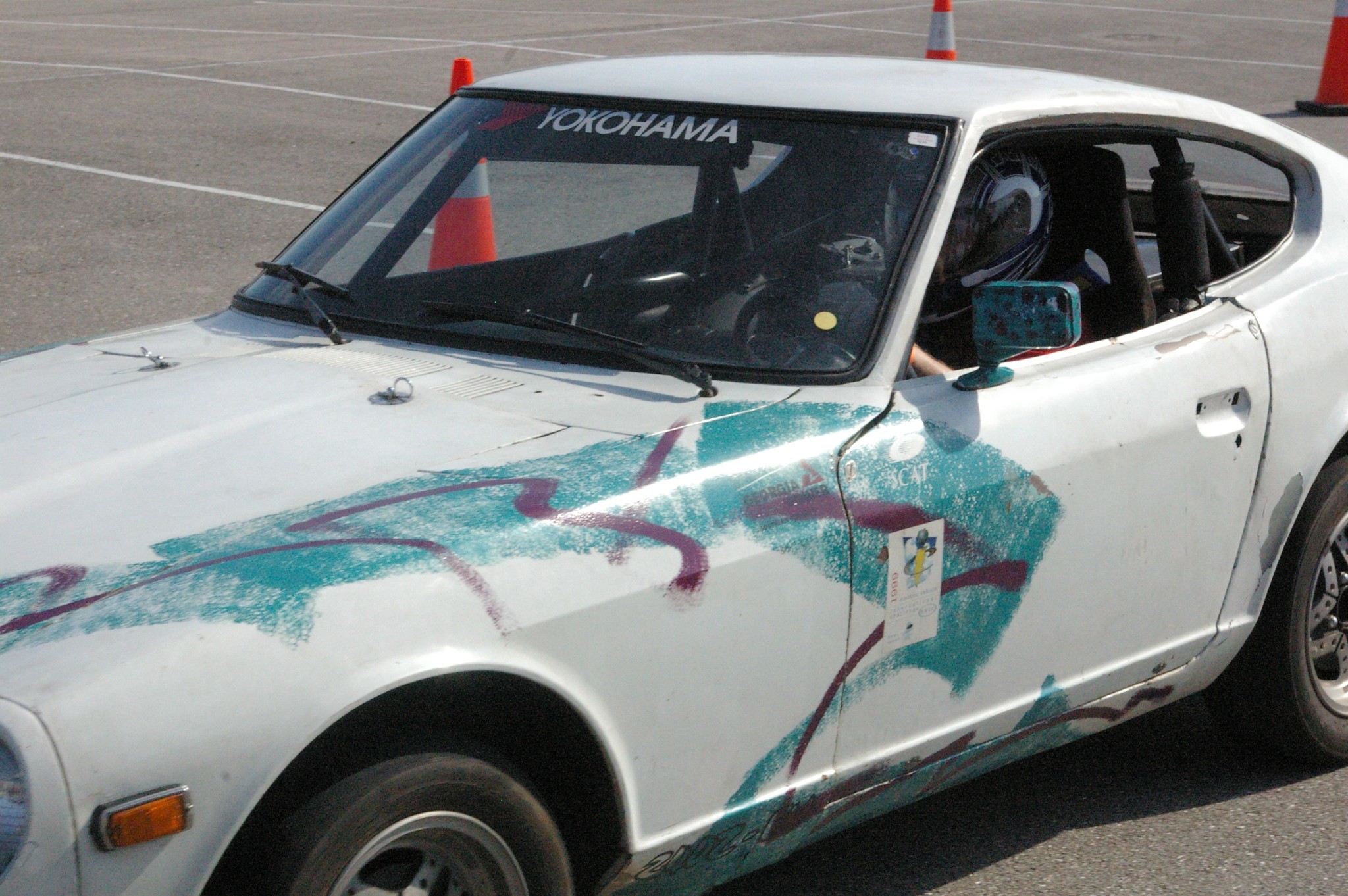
[1034,145,1157,337]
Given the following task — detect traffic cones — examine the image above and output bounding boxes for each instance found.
[430,56,499,273]
[1293,0,1348,117]
[920,0,958,61]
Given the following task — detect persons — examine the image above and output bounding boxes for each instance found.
[760,154,1053,371]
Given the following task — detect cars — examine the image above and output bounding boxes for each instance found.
[2,53,1347,895]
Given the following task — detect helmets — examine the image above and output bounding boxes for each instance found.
[884,147,1055,328]
